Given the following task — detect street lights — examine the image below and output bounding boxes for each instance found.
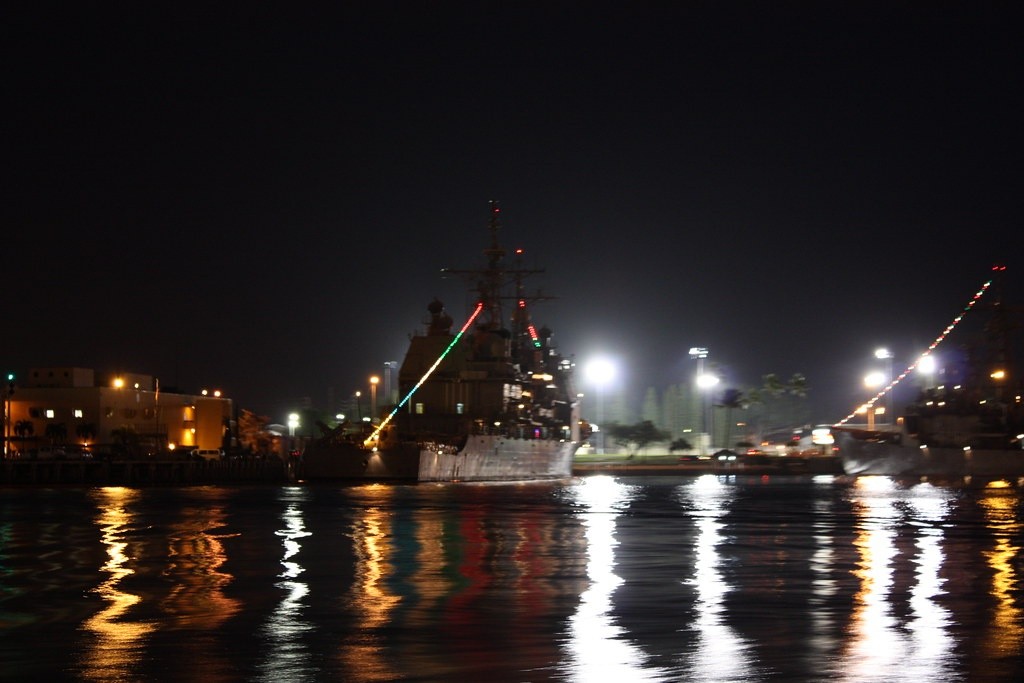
[291,413,299,437]
[355,392,361,421]
[367,376,379,425]
[688,348,708,433]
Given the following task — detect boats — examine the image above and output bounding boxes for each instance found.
[831,369,1024,479]
[290,185,592,483]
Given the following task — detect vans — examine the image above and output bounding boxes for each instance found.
[189,447,222,463]
